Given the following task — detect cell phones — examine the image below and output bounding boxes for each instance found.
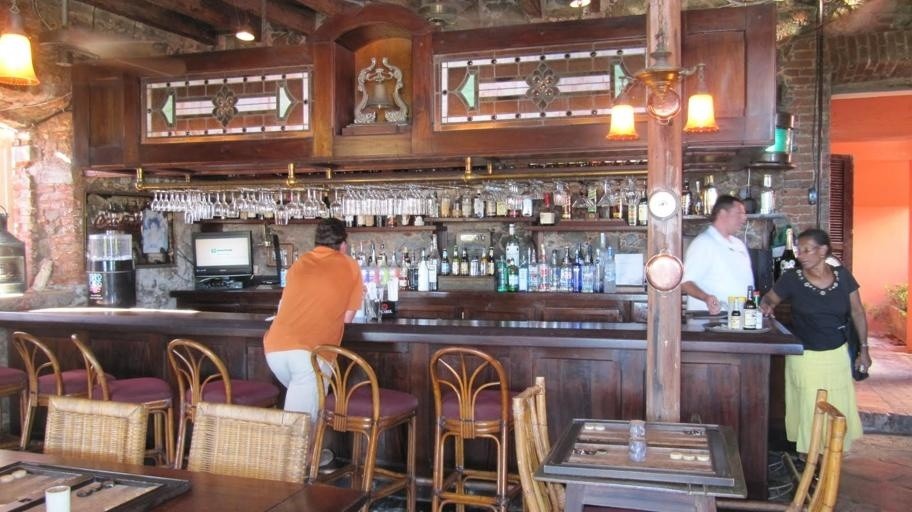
[857,351,867,374]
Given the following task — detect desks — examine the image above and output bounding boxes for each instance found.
[534,419,747,512]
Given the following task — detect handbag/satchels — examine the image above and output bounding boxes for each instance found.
[839,267,869,381]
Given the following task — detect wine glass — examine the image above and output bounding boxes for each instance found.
[148,186,427,231]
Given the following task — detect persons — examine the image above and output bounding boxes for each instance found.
[680,193,755,316]
[262,217,366,426]
[758,227,873,469]
[148,218,162,250]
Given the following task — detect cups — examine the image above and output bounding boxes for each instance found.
[44,486,73,512]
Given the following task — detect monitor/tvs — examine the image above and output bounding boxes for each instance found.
[191,229,254,281]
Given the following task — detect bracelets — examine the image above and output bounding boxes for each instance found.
[859,343,869,347]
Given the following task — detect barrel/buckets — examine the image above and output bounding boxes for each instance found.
[85,230,137,308]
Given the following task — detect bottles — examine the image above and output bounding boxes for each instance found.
[725,285,772,331]
[627,417,650,463]
[277,246,493,292]
[424,173,782,225]
[497,226,616,296]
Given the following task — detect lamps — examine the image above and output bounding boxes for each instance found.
[235,14,255,41]
[0,0,40,86]
[605,0,719,141]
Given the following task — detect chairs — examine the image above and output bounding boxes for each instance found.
[71,333,174,468]
[188,402,311,482]
[512,377,566,512]
[0,367,28,433]
[430,347,529,512]
[167,339,280,469]
[309,344,419,512]
[43,395,148,465]
[11,332,115,450]
[715,389,846,512]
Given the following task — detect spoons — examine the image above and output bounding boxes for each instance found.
[70,479,119,498]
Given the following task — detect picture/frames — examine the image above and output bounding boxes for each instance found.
[267,243,294,267]
[84,191,173,269]
